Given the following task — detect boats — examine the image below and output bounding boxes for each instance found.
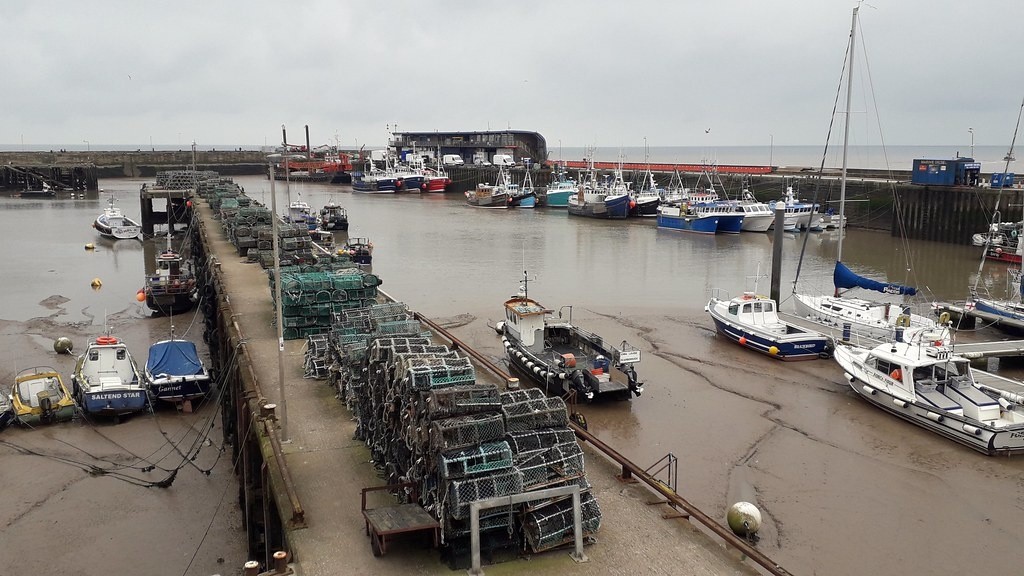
[141,306,212,402]
[267,122,847,247]
[831,317,1024,459]
[70,307,148,417]
[10,365,75,427]
[706,264,832,360]
[342,236,373,266]
[93,194,141,240]
[791,9,951,346]
[20,187,55,196]
[969,297,1024,335]
[144,233,198,309]
[971,233,1024,264]
[501,268,644,402]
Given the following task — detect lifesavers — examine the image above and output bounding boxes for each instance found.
[95,336,118,345]
[896,314,910,328]
[939,312,950,325]
[1011,230,1017,238]
